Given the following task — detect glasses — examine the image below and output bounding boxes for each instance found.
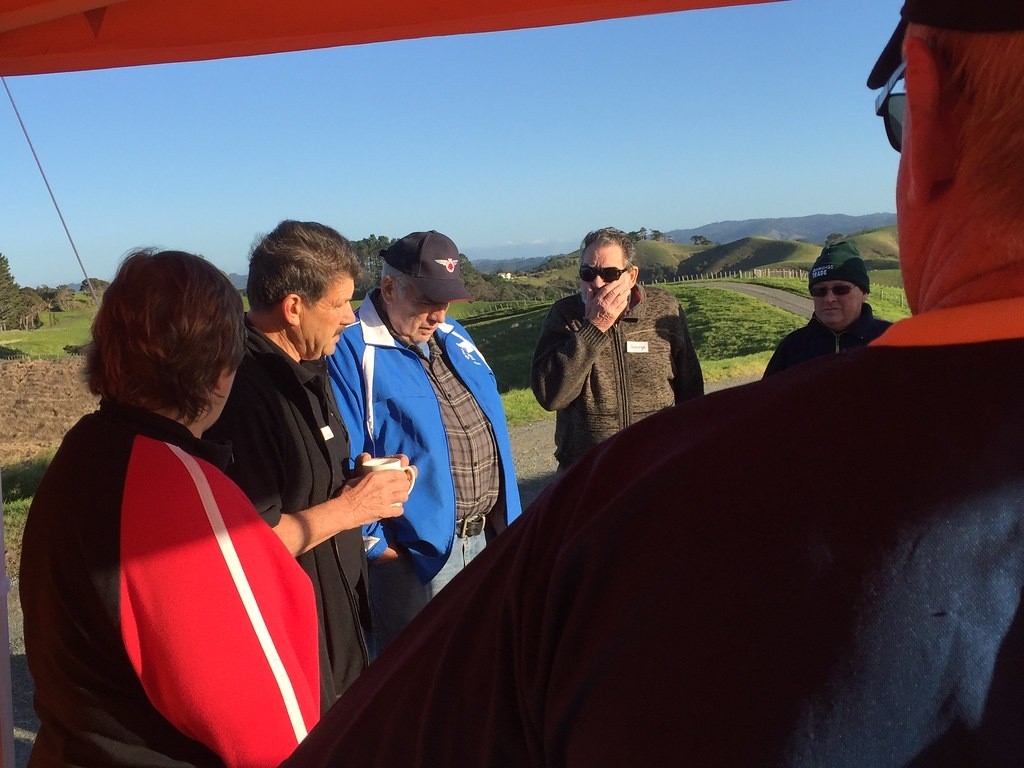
[810,284,856,297]
[579,266,627,283]
[874,62,907,152]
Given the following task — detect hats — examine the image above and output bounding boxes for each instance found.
[809,241,870,293]
[866,0,1024,90]
[379,229,473,304]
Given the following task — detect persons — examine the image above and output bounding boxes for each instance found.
[530,228,705,479]
[329,229,524,658]
[278,0,1023,768]
[762,233,894,378]
[209,225,419,716]
[19,246,320,767]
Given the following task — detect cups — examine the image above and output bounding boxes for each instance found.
[362,457,415,507]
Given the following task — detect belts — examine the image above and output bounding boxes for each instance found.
[454,513,491,538]
